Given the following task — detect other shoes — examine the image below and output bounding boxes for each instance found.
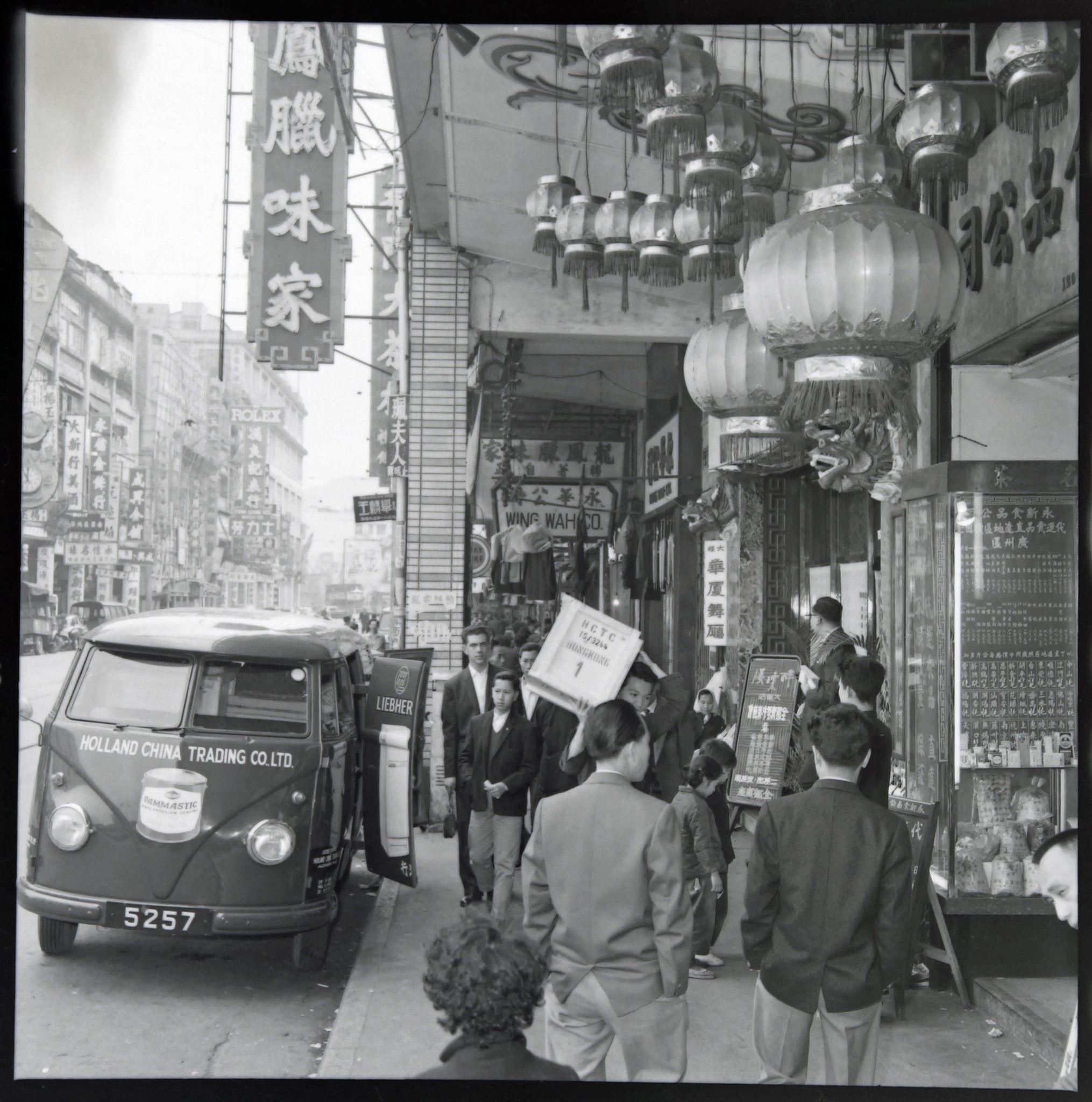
[689,966,715,979]
[461,893,483,906]
[695,952,723,965]
[487,890,493,901]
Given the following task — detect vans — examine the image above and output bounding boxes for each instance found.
[70,601,130,630]
[15,611,435,956]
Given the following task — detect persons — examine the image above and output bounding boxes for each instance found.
[787,656,907,1018]
[350,623,359,632]
[698,739,736,945]
[1031,826,1079,1092]
[457,606,727,865]
[488,640,508,667]
[460,671,541,924]
[787,595,856,793]
[415,914,576,1078]
[361,618,386,657]
[521,698,694,1083]
[671,756,725,978]
[359,609,369,633]
[741,703,916,1084]
[344,615,351,626]
[441,625,521,908]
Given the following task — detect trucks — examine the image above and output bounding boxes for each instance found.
[20,580,65,656]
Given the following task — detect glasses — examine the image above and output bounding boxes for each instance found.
[805,612,823,621]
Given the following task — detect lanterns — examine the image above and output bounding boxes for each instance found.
[981,16,1074,134]
[737,181,971,418]
[683,292,798,469]
[895,86,983,195]
[529,21,787,286]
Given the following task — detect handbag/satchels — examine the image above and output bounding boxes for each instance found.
[443,792,457,838]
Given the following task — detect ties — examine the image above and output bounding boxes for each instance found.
[643,709,655,783]
